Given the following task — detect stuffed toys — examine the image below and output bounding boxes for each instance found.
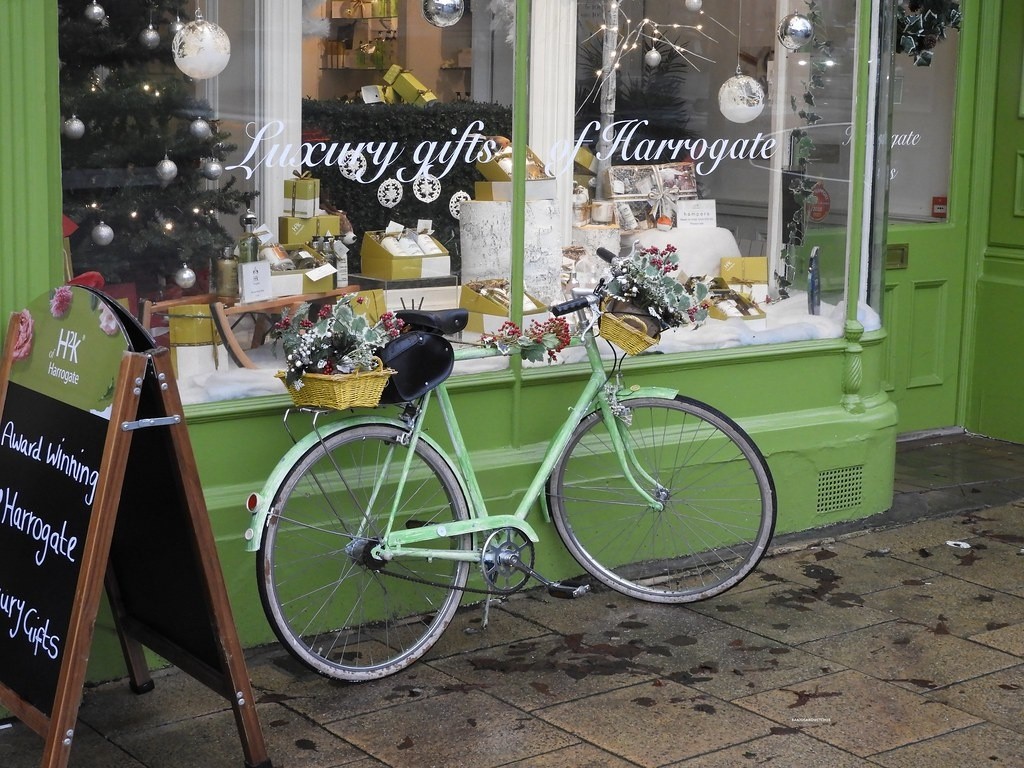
[319,200,358,245]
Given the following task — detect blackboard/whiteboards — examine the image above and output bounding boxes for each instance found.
[0,378,275,743]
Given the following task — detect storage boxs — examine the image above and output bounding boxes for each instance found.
[259,244,334,295]
[361,85,401,104]
[318,215,340,236]
[170,304,221,346]
[360,231,449,258]
[171,346,228,380]
[361,257,450,281]
[600,296,661,356]
[708,294,766,321]
[383,64,438,107]
[709,277,730,291]
[721,257,768,302]
[475,143,557,181]
[459,279,549,317]
[278,216,317,244]
[475,182,557,202]
[284,179,320,219]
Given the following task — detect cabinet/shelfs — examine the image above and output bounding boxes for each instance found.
[318,0,398,103]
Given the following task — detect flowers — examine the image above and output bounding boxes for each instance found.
[464,311,572,336]
[270,291,404,392]
[603,243,720,332]
[471,317,572,364]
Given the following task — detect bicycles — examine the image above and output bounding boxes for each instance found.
[245,247,777,683]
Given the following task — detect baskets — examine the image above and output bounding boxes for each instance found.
[276,353,398,410]
[600,294,661,357]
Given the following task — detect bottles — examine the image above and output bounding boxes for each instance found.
[238,218,258,263]
[290,250,318,270]
[618,201,638,229]
[216,247,239,298]
[240,213,296,271]
